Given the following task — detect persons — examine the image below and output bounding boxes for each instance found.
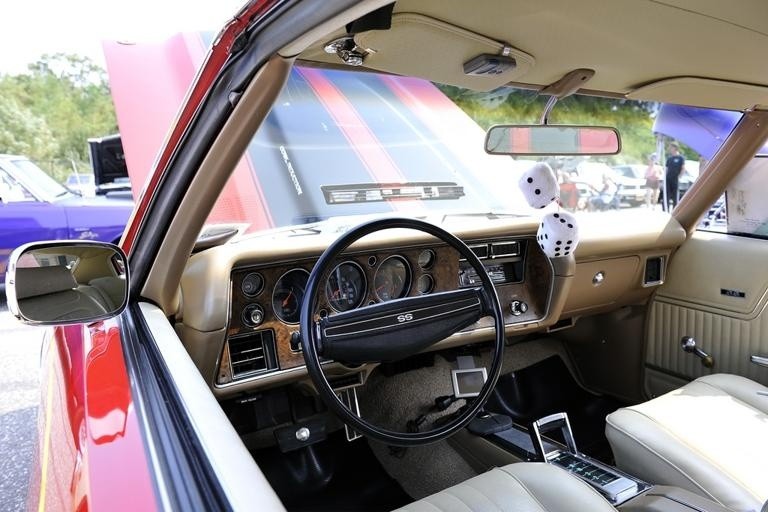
[661,141,687,212]
[558,172,581,211]
[584,172,619,210]
[644,153,662,209]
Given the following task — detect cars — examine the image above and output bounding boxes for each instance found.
[559,158,727,220]
[1,0,766,512]
[1,154,138,295]
[65,134,139,202]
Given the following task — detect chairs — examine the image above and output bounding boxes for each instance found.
[16,266,123,321]
